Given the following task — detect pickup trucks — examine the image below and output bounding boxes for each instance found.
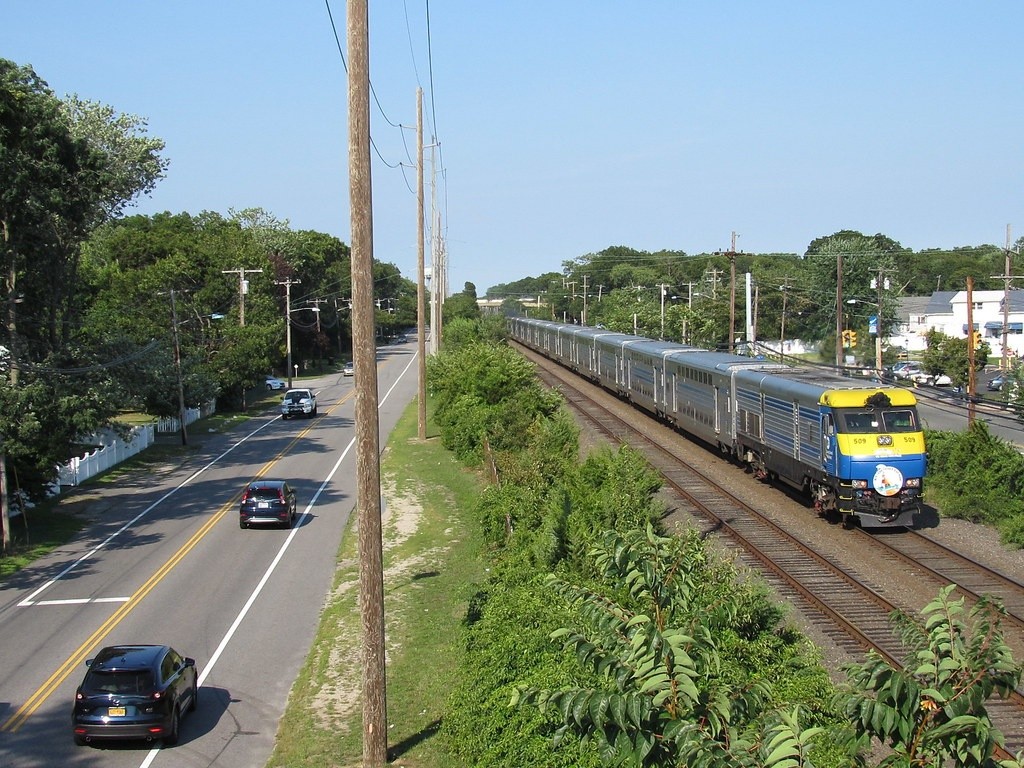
[278,388,319,419]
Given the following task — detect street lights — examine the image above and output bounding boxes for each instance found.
[905,339,909,368]
[845,300,882,380]
[588,295,601,304]
[287,308,323,392]
[693,293,716,342]
[380,307,394,344]
[174,313,224,447]
[573,294,587,327]
[671,296,691,345]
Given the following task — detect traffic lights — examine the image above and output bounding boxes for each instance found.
[843,331,851,348]
[849,329,859,349]
[973,331,983,350]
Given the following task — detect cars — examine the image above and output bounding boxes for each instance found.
[238,480,298,530]
[343,362,354,376]
[265,375,286,392]
[69,644,199,748]
[879,361,927,382]
[397,334,407,344]
[911,371,952,387]
[986,373,1017,392]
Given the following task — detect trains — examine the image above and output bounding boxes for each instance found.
[500,314,926,529]
